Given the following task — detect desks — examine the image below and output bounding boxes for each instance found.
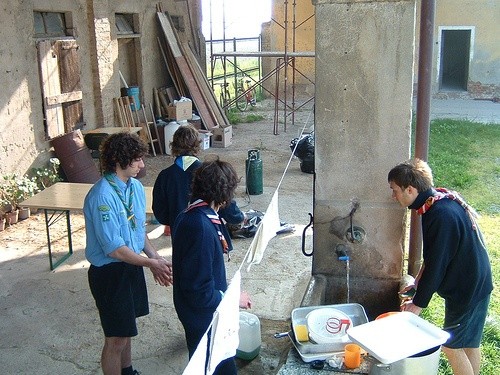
[81,126,142,135]
[19,183,154,271]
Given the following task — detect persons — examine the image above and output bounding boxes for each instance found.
[152,125,245,254]
[388,159,493,375]
[83,133,173,375]
[173,160,251,361]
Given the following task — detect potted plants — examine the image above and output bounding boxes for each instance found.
[0,174,22,230]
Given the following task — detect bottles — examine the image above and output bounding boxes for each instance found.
[235,311,262,361]
[162,117,181,156]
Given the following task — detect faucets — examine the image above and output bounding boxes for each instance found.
[338,256,350,273]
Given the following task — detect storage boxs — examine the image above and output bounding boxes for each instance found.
[197,129,213,150]
[211,125,232,148]
[167,101,192,122]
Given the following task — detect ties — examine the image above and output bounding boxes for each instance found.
[174,155,200,172]
[184,199,230,264]
[417,187,482,230]
[104,171,136,232]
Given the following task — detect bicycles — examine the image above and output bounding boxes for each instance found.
[216,83,230,107]
[236,77,257,112]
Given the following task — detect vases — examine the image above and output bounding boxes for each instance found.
[30,208,38,214]
[19,208,31,220]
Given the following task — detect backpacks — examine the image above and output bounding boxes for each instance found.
[225,209,264,238]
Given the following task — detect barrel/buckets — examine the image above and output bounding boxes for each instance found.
[121,86,140,111]
[49,128,101,185]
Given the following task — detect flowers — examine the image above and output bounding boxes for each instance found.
[15,157,64,201]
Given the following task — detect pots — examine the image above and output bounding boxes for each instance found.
[360,345,443,375]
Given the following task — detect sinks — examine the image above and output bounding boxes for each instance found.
[299,273,411,321]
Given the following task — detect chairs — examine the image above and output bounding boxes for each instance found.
[84,132,110,178]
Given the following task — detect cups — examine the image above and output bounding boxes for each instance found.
[344,343,360,368]
